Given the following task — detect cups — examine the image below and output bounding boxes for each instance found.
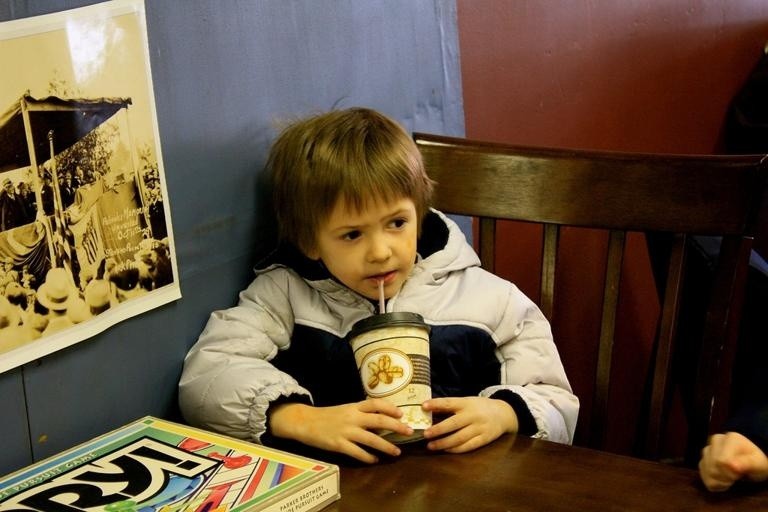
[345,310,433,446]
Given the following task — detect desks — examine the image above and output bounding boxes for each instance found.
[320,410,766,512]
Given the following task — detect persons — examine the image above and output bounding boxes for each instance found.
[177,106,581,462]
[0,165,170,357]
[697,430,768,494]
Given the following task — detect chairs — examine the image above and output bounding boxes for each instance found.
[409,126,768,460]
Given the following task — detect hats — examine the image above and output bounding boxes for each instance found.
[2,178,11,187]
[36,268,79,311]
[85,261,140,307]
[17,182,32,194]
[2,257,15,264]
